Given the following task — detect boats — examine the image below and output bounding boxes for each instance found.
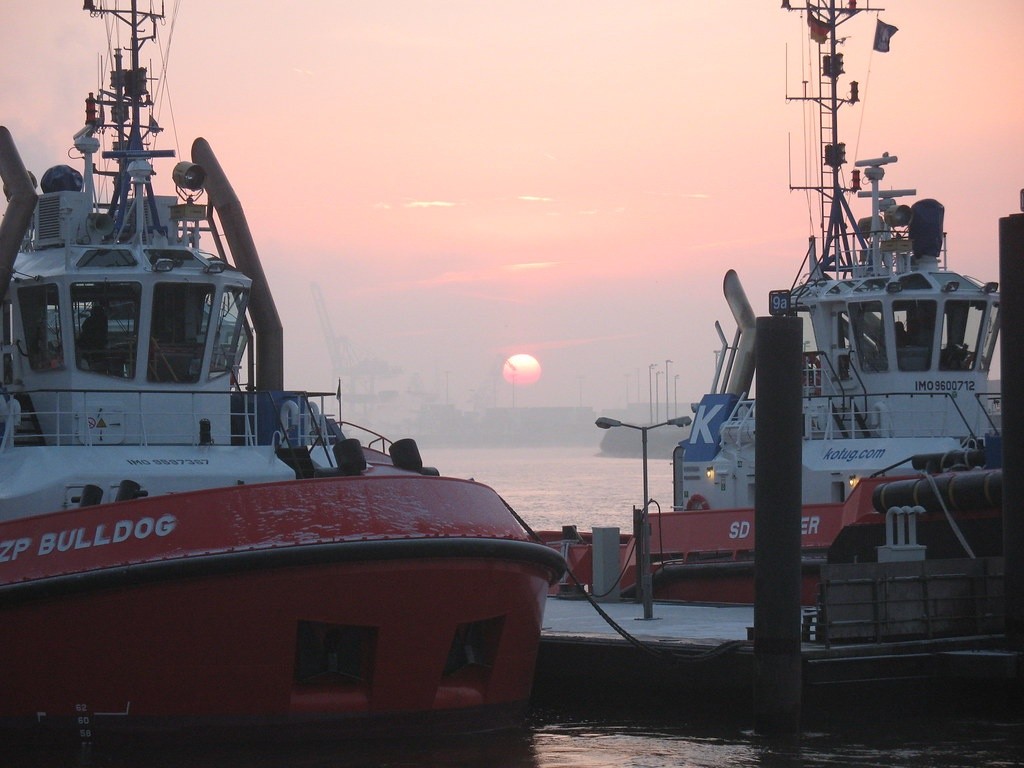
[531,1,1000,611]
[0,1,568,728]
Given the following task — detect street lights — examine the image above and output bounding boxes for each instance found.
[665,359,673,421]
[648,363,657,424]
[655,370,664,424]
[594,411,693,622]
[675,375,680,418]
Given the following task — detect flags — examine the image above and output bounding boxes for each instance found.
[810,16,830,44]
[873,19,898,52]
